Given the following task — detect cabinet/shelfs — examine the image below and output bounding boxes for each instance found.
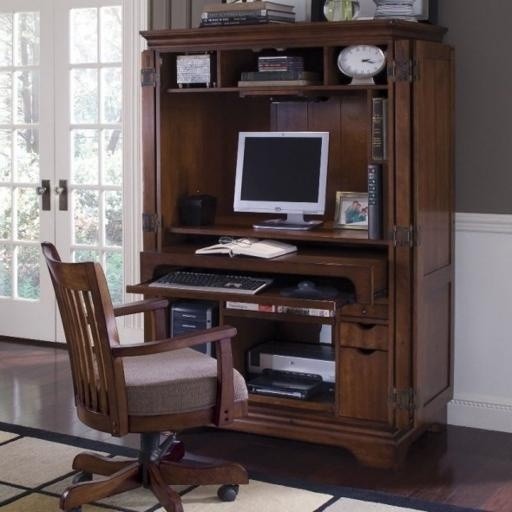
[126,16,455,470]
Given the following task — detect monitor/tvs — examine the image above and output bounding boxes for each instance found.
[234,131,329,231]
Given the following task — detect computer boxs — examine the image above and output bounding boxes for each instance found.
[169,301,220,356]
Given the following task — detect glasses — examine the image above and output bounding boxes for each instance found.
[219,237,252,248]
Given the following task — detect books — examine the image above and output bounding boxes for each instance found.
[198,1,296,28]
[195,238,298,259]
[238,56,323,86]
[225,301,335,319]
[372,97,387,161]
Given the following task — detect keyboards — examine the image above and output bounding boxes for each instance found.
[148,270,273,296]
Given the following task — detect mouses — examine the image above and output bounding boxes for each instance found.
[298,281,315,290]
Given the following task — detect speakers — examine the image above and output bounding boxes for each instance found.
[180,195,216,226]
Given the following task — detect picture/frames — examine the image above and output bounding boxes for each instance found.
[332,188,371,231]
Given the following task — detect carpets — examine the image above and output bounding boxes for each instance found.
[0,420,488,511]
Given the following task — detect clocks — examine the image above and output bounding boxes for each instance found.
[337,43,386,86]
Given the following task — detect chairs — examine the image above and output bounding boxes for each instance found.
[38,241,250,512]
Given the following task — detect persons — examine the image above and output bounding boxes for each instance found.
[344,200,366,224]
